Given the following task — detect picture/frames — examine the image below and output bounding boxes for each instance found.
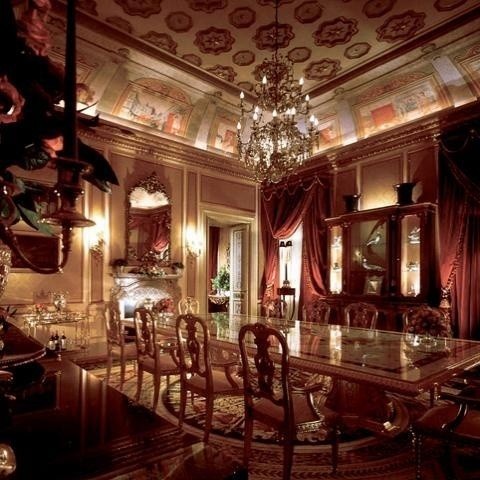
[9,230,63,274]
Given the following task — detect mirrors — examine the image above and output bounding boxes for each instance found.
[125,170,172,266]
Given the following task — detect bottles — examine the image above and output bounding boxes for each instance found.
[61,329,67,350]
[55,330,60,351]
[50,331,55,352]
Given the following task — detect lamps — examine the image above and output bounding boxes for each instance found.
[236,0,319,184]
[176,314,245,443]
[279,241,292,288]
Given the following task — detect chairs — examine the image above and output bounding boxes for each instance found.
[133,307,193,413]
[178,296,200,316]
[102,301,145,392]
[403,303,447,410]
[344,302,378,330]
[302,299,331,323]
[410,371,479,480]
[262,299,287,318]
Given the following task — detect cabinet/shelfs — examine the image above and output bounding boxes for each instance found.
[318,202,438,334]
